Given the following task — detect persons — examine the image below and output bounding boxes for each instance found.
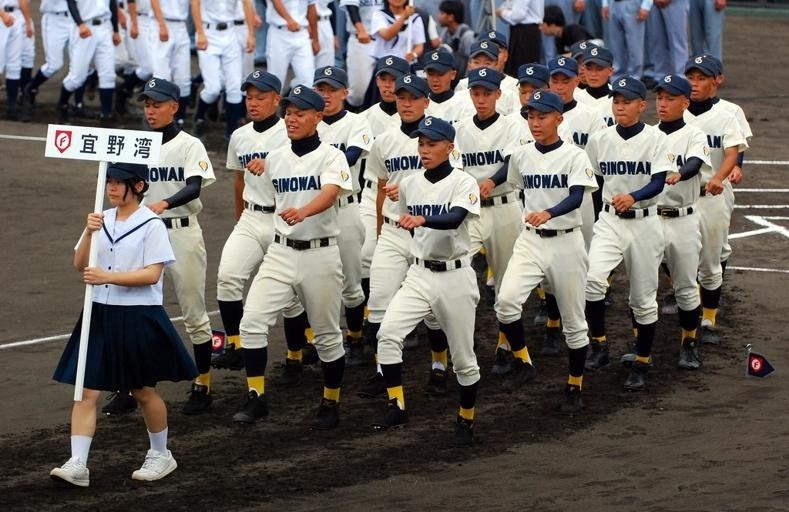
[315,0,339,69]
[264,0,320,93]
[190,0,254,144]
[211,69,305,388]
[55,0,121,127]
[507,64,572,356]
[600,0,654,85]
[242,2,263,78]
[584,75,681,393]
[663,53,749,348]
[645,0,690,92]
[21,15,35,89]
[456,29,518,266]
[117,0,153,113]
[358,72,463,392]
[115,6,128,75]
[51,162,200,488]
[539,0,584,62]
[148,0,191,127]
[370,115,482,445]
[547,56,597,250]
[338,0,385,111]
[414,7,442,60]
[620,73,705,370]
[422,50,467,125]
[231,84,352,432]
[436,0,476,47]
[364,0,427,105]
[0,1,32,119]
[456,42,519,302]
[303,66,373,369]
[570,41,600,92]
[574,48,616,130]
[22,0,94,122]
[539,4,593,56]
[496,0,544,70]
[453,65,524,376]
[661,53,753,315]
[690,0,727,62]
[102,77,214,415]
[358,54,410,327]
[494,90,599,417]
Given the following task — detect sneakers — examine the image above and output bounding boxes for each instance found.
[311,398,340,430]
[346,335,364,366]
[181,383,213,416]
[23,83,38,110]
[101,390,137,417]
[534,299,547,325]
[278,357,303,386]
[678,336,700,370]
[356,372,385,398]
[232,389,269,424]
[281,344,317,367]
[623,360,653,391]
[99,113,114,127]
[132,449,178,482]
[71,104,94,119]
[426,368,448,395]
[381,397,409,427]
[500,358,536,391]
[585,340,610,371]
[55,105,71,125]
[50,457,90,488]
[560,382,583,415]
[211,342,244,370]
[451,415,475,445]
[190,115,204,138]
[620,342,653,368]
[700,324,721,345]
[487,348,511,383]
[540,324,561,355]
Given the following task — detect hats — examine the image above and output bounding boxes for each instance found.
[570,40,597,59]
[582,45,614,67]
[279,84,326,111]
[312,65,348,89]
[683,55,717,79]
[520,89,563,114]
[652,72,691,98]
[409,115,456,144]
[135,77,181,102]
[470,39,498,60]
[375,54,409,78]
[422,51,456,73]
[391,72,429,98]
[240,69,281,94]
[478,29,507,48]
[516,63,550,87]
[607,76,646,100]
[466,67,500,90]
[702,53,723,75]
[549,56,578,78]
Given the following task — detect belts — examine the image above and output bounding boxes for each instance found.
[89,18,107,25]
[162,216,189,229]
[273,234,329,251]
[480,195,508,208]
[699,186,712,197]
[305,15,327,21]
[339,195,353,206]
[4,6,17,11]
[416,257,461,272]
[525,225,574,238]
[53,11,70,16]
[384,217,400,226]
[657,206,694,218]
[205,23,228,31]
[234,20,245,26]
[244,201,276,213]
[273,25,288,30]
[605,203,649,220]
[366,180,376,189]
